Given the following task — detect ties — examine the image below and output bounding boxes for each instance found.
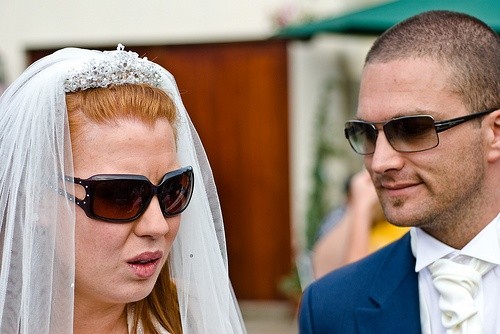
[426,256,497,334]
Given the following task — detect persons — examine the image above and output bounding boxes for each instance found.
[1,43,249,334]
[296,10,500,334]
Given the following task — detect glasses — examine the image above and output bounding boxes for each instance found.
[344,108,499,156]
[39,165,195,223]
